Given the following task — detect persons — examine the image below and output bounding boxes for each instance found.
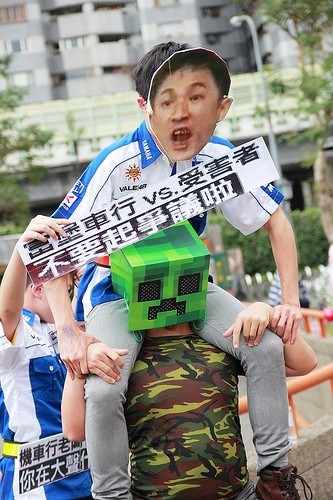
[27,42,318,500]
[0,214,96,499]
[148,46,233,162]
[60,302,318,500]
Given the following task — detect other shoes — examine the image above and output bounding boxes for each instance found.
[255,461,313,500]
